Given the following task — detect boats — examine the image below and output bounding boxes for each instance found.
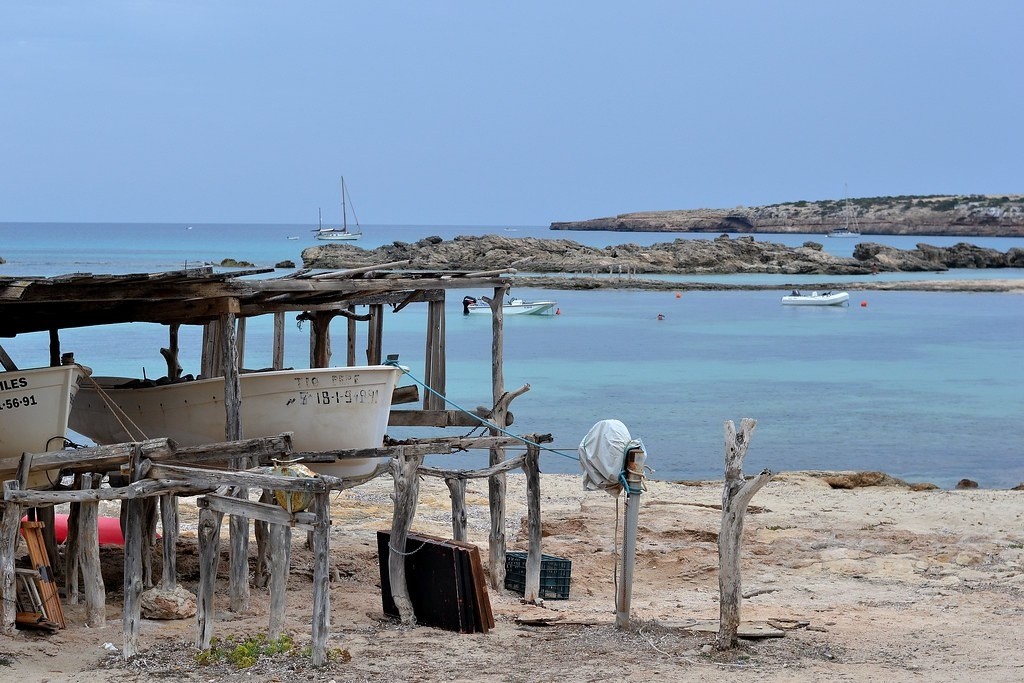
[0,351,93,496]
[65,366,412,480]
[781,291,849,307]
[462,295,558,315]
[186,226,192,229]
[288,235,299,240]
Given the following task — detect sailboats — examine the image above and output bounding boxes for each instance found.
[826,179,860,237]
[311,207,322,231]
[314,175,363,240]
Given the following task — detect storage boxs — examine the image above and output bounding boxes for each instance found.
[503,552,572,601]
